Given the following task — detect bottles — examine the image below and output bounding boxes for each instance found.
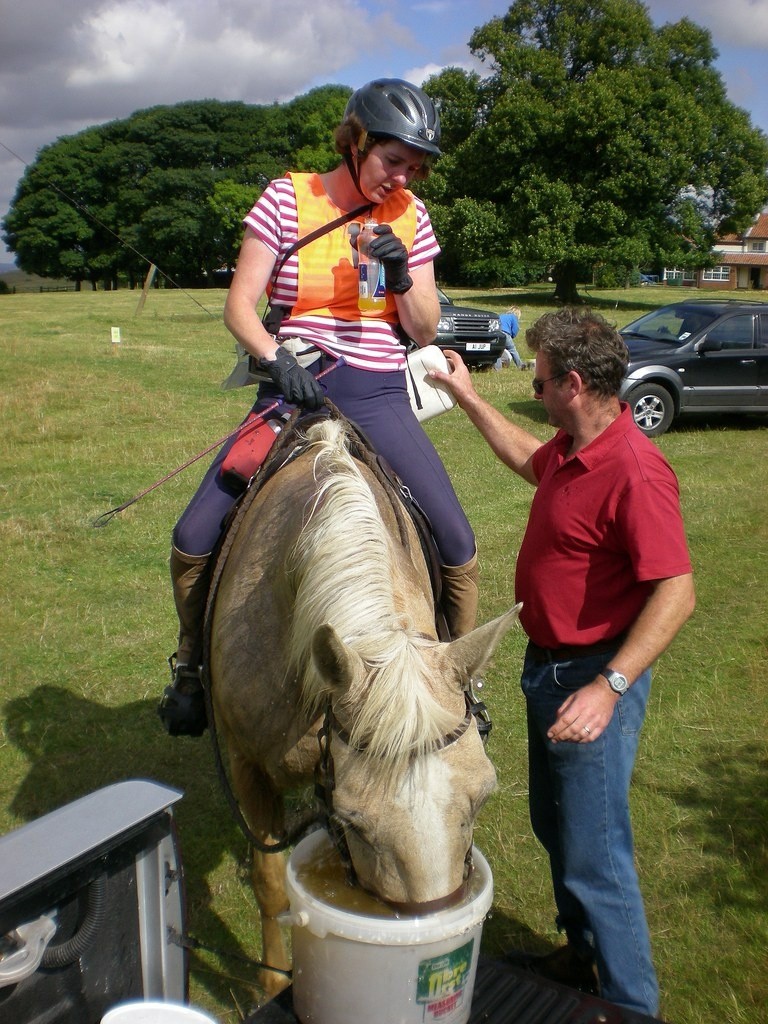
[357,219,387,311]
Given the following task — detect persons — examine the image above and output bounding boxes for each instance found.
[427,302,697,1019]
[495,306,528,371]
[156,79,492,748]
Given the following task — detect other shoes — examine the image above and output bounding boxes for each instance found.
[522,945,599,986]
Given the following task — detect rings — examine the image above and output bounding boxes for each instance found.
[584,726,591,735]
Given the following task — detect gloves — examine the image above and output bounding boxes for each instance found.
[348,221,414,293]
[255,346,324,411]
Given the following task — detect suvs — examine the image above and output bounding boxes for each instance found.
[613,297,768,441]
[403,285,506,372]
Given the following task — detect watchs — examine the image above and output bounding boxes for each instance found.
[597,666,631,696]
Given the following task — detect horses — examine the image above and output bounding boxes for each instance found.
[208,419,524,1017]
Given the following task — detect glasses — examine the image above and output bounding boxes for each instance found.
[532,370,570,394]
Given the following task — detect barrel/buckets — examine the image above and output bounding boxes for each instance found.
[100,1001,215,1024]
[278,824,495,1024]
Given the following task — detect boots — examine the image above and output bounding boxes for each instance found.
[436,540,493,733]
[168,541,213,730]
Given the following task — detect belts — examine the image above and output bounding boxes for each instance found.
[525,640,617,663]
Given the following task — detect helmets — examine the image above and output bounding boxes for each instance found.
[342,76,442,155]
